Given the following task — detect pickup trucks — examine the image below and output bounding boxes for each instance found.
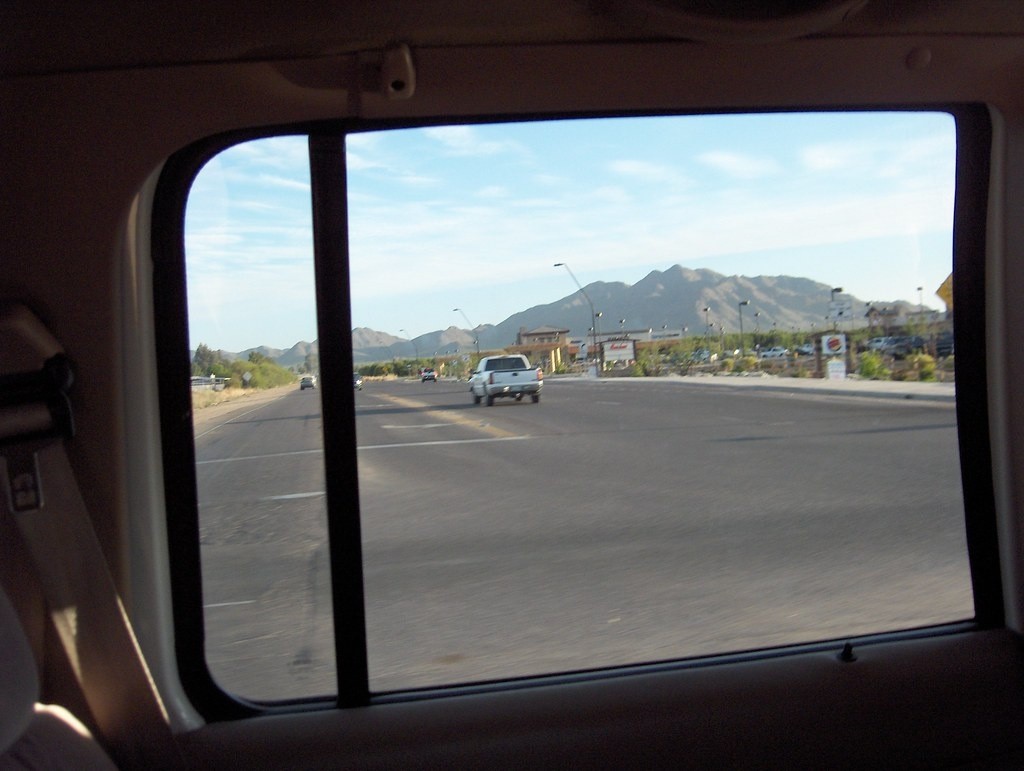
[468,353,544,405]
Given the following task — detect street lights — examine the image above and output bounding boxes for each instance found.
[399,329,420,364]
[453,308,480,362]
[831,287,843,332]
[739,300,750,357]
[554,262,600,377]
[703,307,711,350]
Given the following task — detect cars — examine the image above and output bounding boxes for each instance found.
[353,371,364,390]
[421,368,438,383]
[760,345,790,357]
[798,333,954,359]
[300,376,316,390]
